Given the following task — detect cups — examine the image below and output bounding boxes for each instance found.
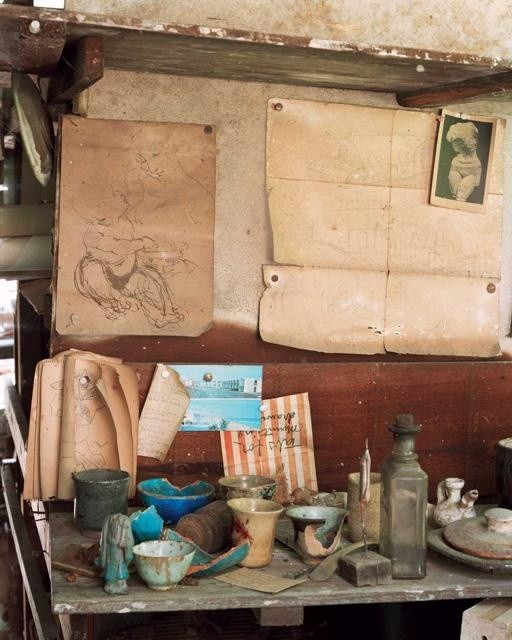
[70,467,130,530]
[286,506,350,566]
[226,497,284,569]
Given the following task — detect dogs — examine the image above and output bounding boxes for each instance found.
[444,120,482,202]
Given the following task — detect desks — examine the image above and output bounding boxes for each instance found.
[46,507,511,640]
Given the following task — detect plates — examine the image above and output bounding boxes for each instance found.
[426,529,511,574]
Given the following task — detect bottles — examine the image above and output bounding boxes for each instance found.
[379,414,429,580]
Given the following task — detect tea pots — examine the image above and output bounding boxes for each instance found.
[432,478,479,528]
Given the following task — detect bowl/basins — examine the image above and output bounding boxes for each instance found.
[132,538,197,591]
[138,477,215,524]
[217,474,278,504]
